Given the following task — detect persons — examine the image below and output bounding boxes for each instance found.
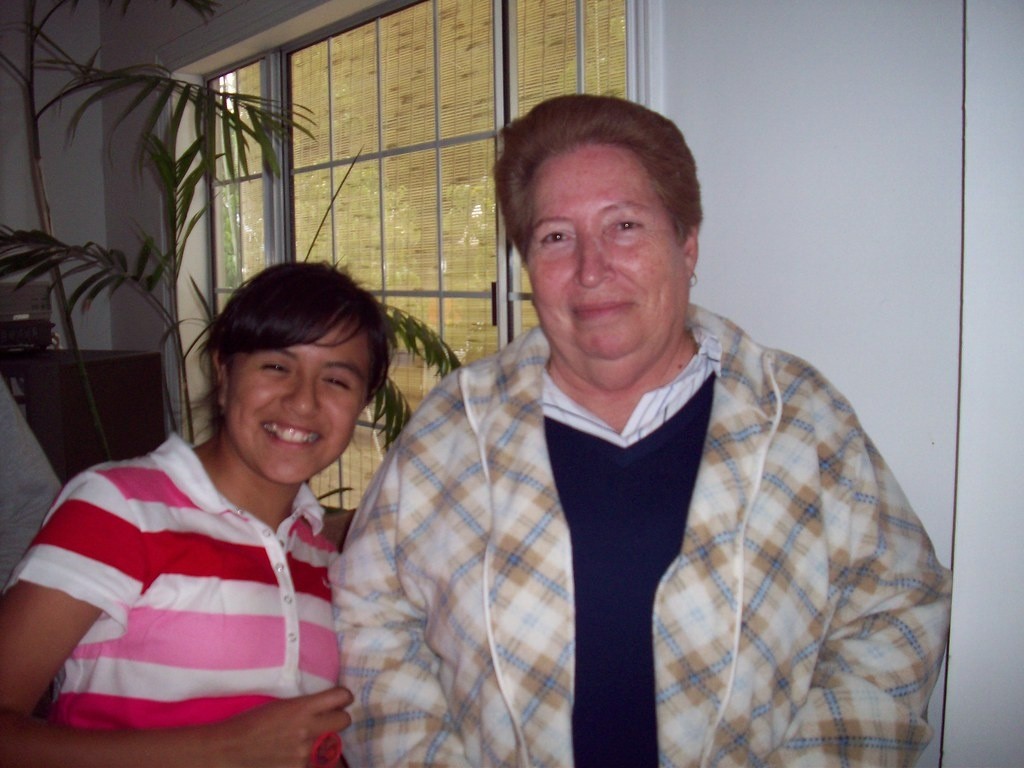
[334,95,956,768]
[0,262,390,768]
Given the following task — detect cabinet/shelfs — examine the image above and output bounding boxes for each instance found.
[0,348,168,489]
